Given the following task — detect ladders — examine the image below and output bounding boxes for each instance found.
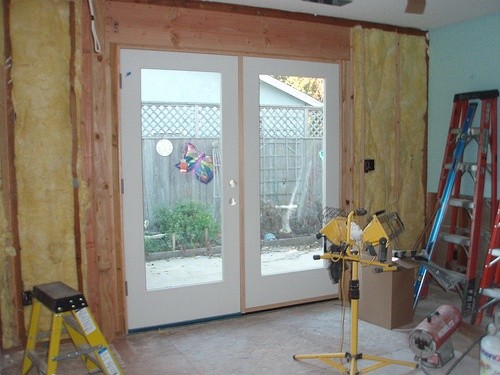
[418,89,500,312]
[21,280,124,375]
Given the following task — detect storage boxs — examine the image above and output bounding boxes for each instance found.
[358,257,415,330]
